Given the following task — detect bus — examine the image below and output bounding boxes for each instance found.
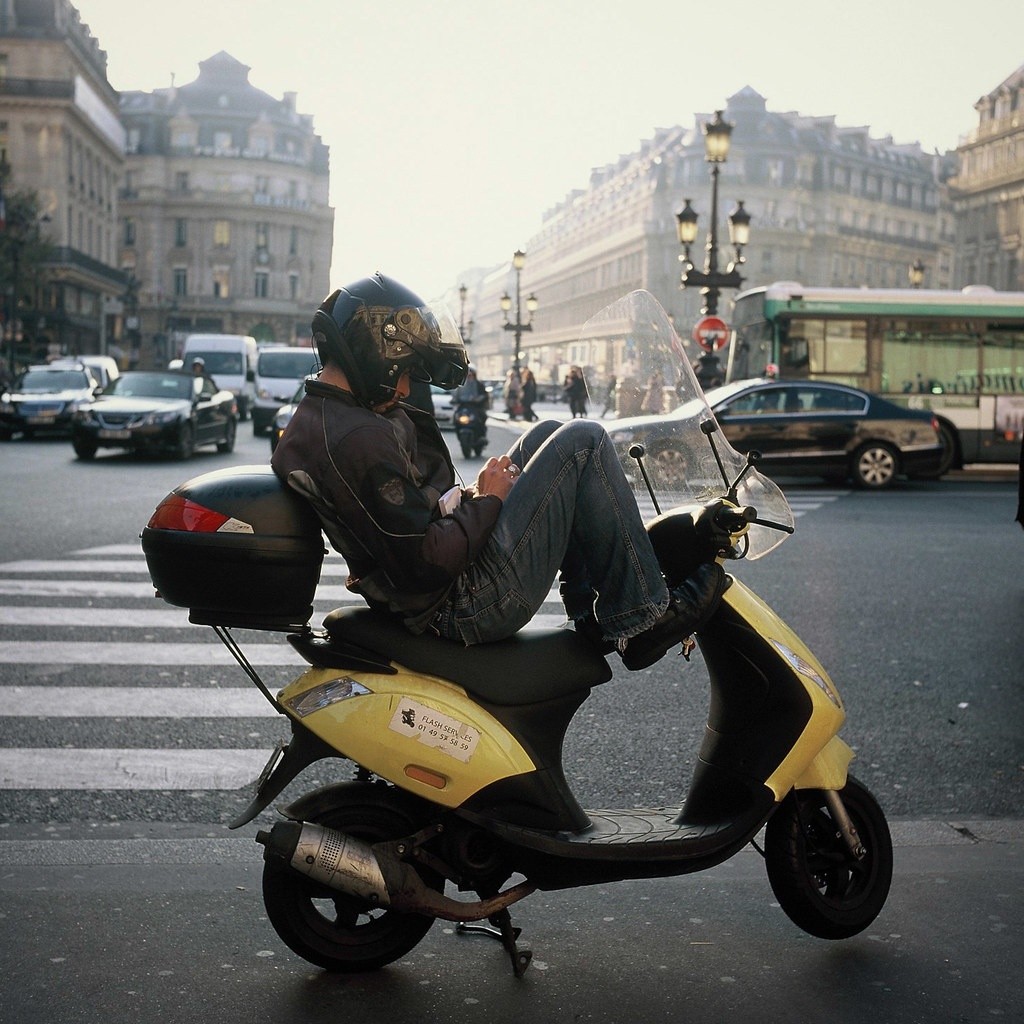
[725,279,1024,464]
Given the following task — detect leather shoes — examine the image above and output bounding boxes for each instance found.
[574,617,617,657]
[614,561,725,671]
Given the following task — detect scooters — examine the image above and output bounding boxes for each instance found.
[139,290,893,983]
[447,377,489,460]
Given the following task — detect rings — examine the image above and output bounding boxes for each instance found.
[507,466,516,474]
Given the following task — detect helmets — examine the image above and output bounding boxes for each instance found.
[192,357,206,368]
[311,269,471,413]
[468,366,476,376]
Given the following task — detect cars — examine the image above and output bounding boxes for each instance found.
[269,373,319,457]
[0,365,100,441]
[426,381,461,432]
[72,368,239,463]
[591,379,955,493]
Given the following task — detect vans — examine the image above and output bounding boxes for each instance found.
[177,334,259,421]
[251,347,320,436]
[62,355,122,395]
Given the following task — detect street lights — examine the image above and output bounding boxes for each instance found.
[498,250,539,370]
[448,283,476,348]
[673,110,755,396]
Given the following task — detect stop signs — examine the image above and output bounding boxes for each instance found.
[694,317,730,354]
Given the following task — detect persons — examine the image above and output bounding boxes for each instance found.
[503,369,540,421]
[641,370,665,415]
[451,367,488,441]
[266,270,729,672]
[600,374,617,418]
[561,369,592,418]
[191,356,205,374]
